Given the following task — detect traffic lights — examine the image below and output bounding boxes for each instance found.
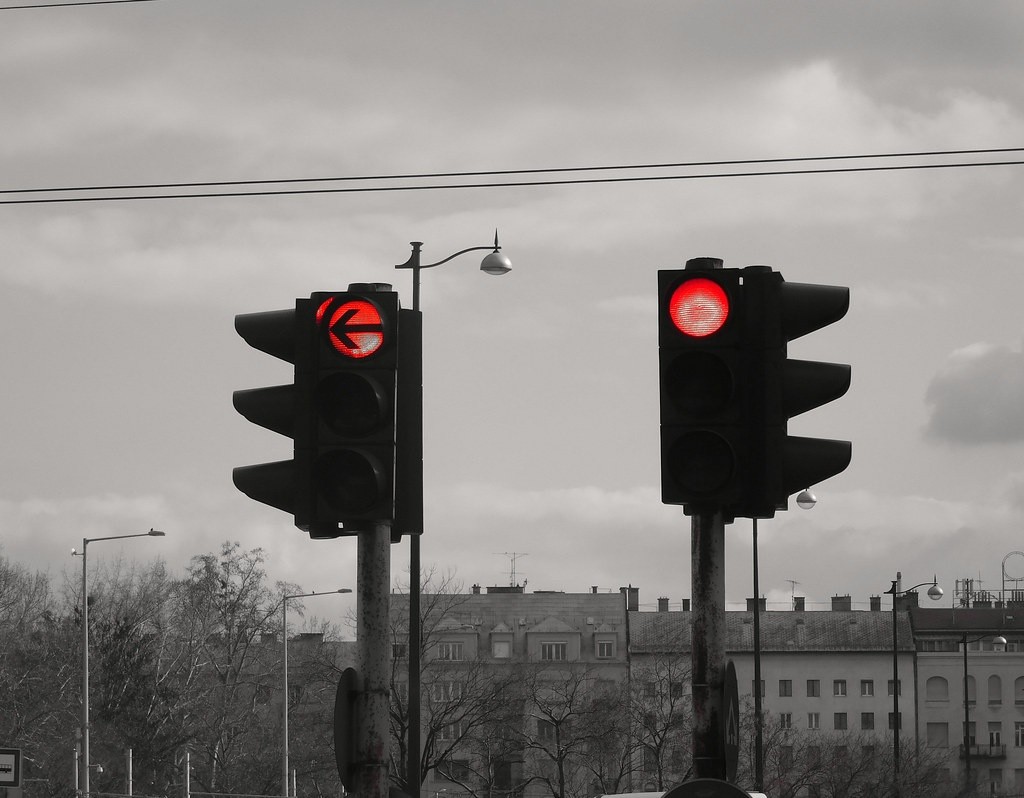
[233,300,316,533]
[305,290,397,524]
[737,265,853,518]
[657,268,744,508]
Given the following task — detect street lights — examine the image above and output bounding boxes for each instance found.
[77,528,167,797]
[752,489,819,798]
[279,589,355,798]
[957,626,1007,798]
[395,234,516,797]
[883,573,945,798]
